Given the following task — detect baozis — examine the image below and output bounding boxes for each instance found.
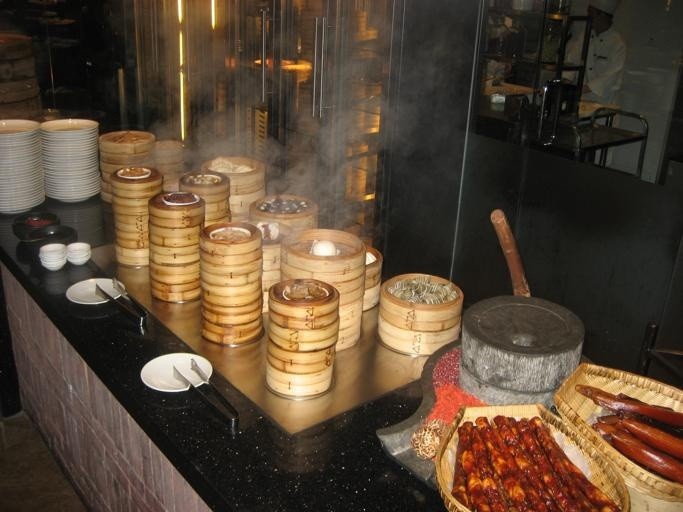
[313,241,337,256]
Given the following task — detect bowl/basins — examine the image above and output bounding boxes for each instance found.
[11,212,92,271]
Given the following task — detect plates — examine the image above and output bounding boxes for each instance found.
[140,353,213,394]
[66,278,128,305]
[0,119,103,215]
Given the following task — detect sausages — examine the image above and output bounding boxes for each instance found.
[452,384,683,512]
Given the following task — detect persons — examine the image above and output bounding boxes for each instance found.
[565,1,626,168]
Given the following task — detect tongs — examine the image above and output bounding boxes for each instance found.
[96,275,151,328]
[173,359,243,428]
